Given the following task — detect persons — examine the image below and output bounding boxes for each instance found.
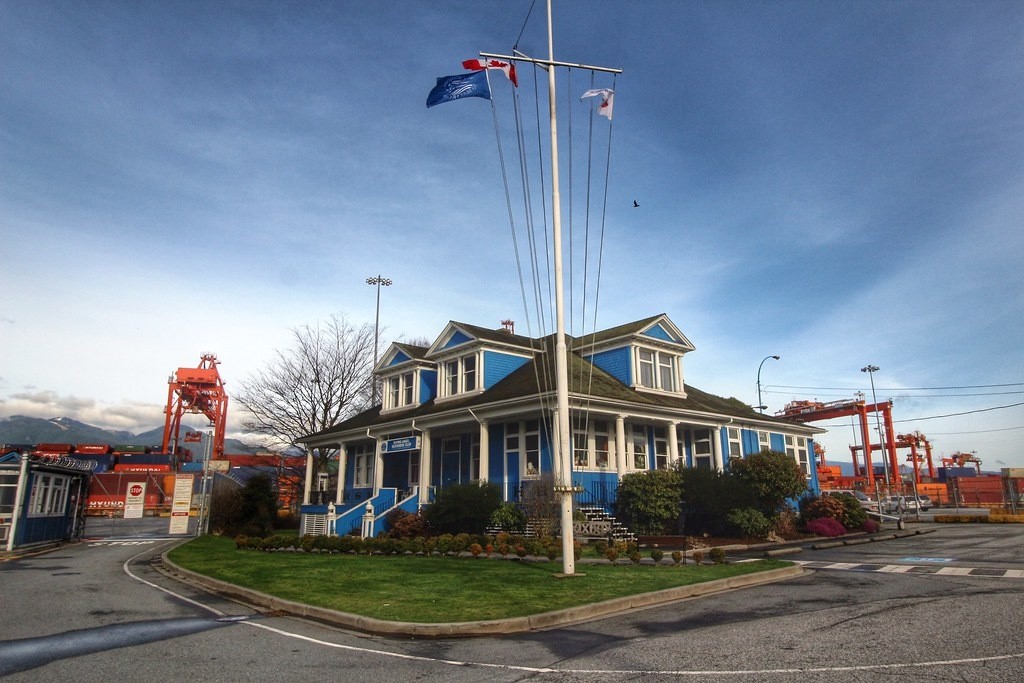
[64,496,82,539]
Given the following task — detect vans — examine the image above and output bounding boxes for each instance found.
[877,495,921,514]
[905,494,933,511]
[820,490,877,512]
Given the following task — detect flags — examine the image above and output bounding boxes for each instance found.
[581,88,614,121]
[462,59,519,87]
[426,68,493,108]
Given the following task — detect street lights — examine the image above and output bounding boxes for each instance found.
[861,364,893,514]
[364,274,393,409]
[758,355,780,415]
